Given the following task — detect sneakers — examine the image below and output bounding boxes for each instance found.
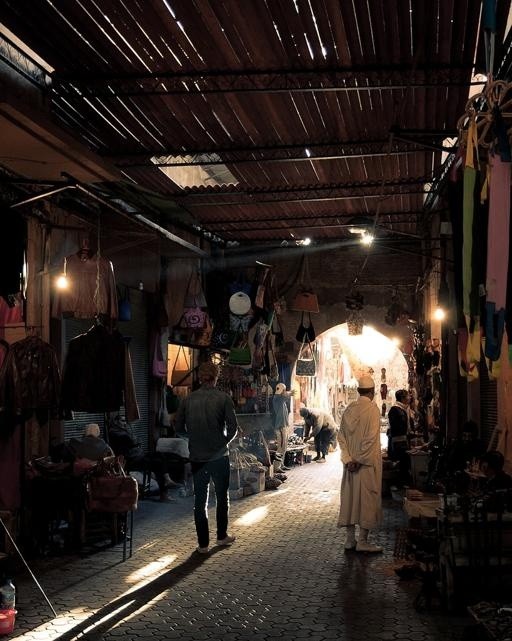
[196,546,209,554]
[355,542,384,552]
[217,536,235,546]
[344,540,357,550]
[312,457,326,463]
[159,479,184,502]
[276,467,290,473]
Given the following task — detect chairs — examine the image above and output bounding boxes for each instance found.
[69,455,125,542]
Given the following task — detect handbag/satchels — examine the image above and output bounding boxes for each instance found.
[272,332,284,346]
[157,384,177,429]
[183,292,208,308]
[170,310,214,348]
[295,322,316,344]
[296,358,315,376]
[292,292,319,314]
[229,347,251,365]
[153,359,168,377]
[271,363,279,379]
[255,284,287,316]
[171,369,192,386]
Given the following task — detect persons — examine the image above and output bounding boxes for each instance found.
[111,414,187,505]
[172,362,241,555]
[381,390,413,508]
[271,383,297,474]
[52,422,134,546]
[337,375,386,553]
[298,407,339,464]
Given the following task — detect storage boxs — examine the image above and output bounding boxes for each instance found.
[239,470,266,492]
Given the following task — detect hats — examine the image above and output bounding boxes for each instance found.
[275,383,287,394]
[358,375,375,389]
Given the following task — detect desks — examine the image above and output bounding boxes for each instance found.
[434,506,511,612]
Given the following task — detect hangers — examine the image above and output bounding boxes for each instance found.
[93,313,103,327]
[25,326,39,344]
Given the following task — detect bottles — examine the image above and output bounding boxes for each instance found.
[1,578,15,610]
